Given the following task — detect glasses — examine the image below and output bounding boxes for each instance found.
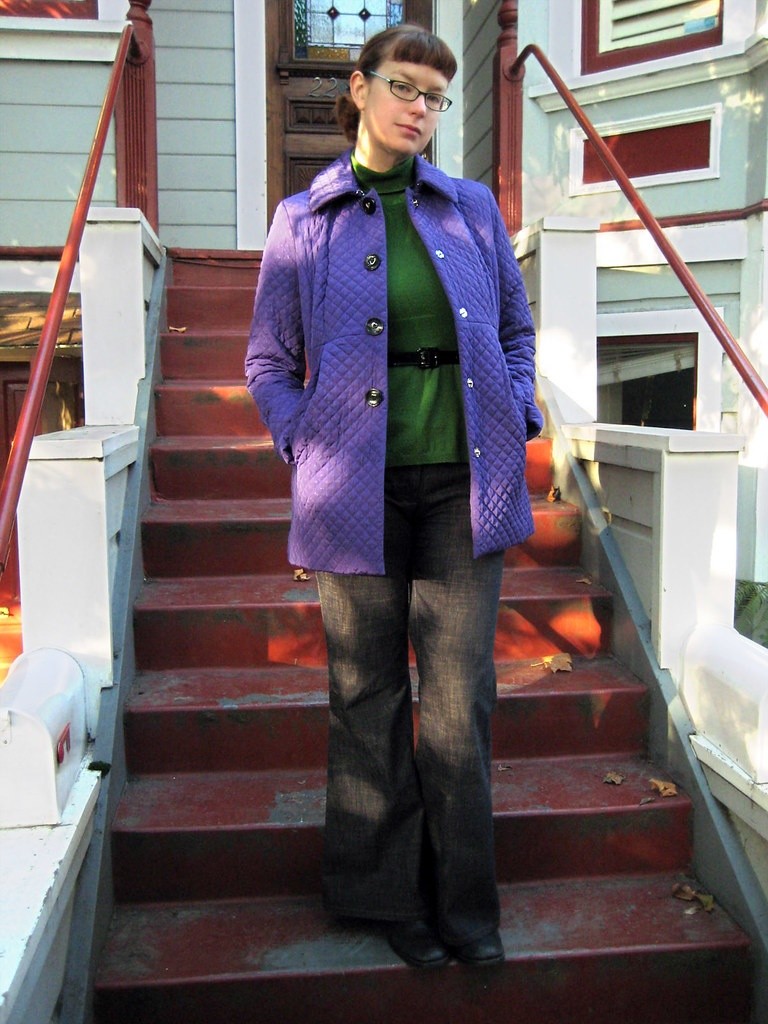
[360,69,452,111]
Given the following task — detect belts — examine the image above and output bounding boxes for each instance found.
[388,348,460,369]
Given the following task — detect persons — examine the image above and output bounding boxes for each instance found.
[244,23,543,969]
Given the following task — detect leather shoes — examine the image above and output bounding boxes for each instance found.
[436,915,504,970]
[379,915,447,966]
